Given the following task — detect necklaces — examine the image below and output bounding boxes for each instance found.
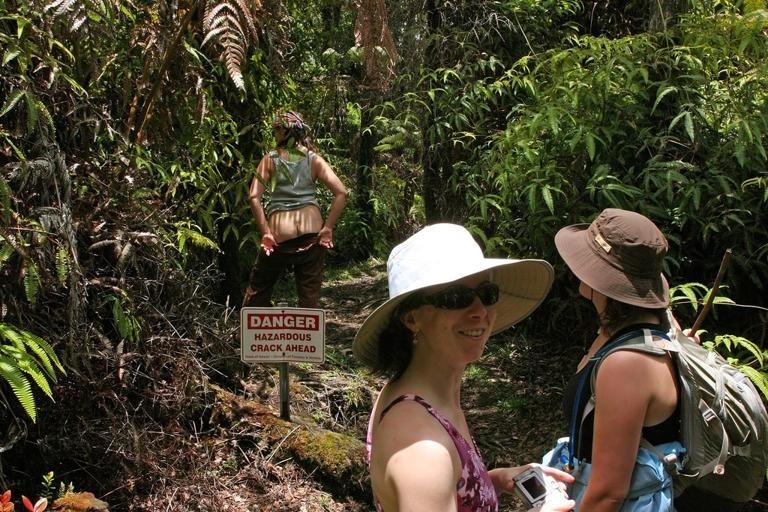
[584,349,592,357]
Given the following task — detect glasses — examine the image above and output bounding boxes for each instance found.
[421,280,500,308]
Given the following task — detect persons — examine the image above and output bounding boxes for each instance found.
[542,208,701,512]
[242,110,349,308]
[353,223,576,512]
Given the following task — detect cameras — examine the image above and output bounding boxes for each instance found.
[513,465,569,510]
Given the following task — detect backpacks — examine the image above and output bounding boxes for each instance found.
[589,307,768,503]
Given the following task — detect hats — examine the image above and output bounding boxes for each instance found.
[555,208,670,310]
[351,223,553,375]
